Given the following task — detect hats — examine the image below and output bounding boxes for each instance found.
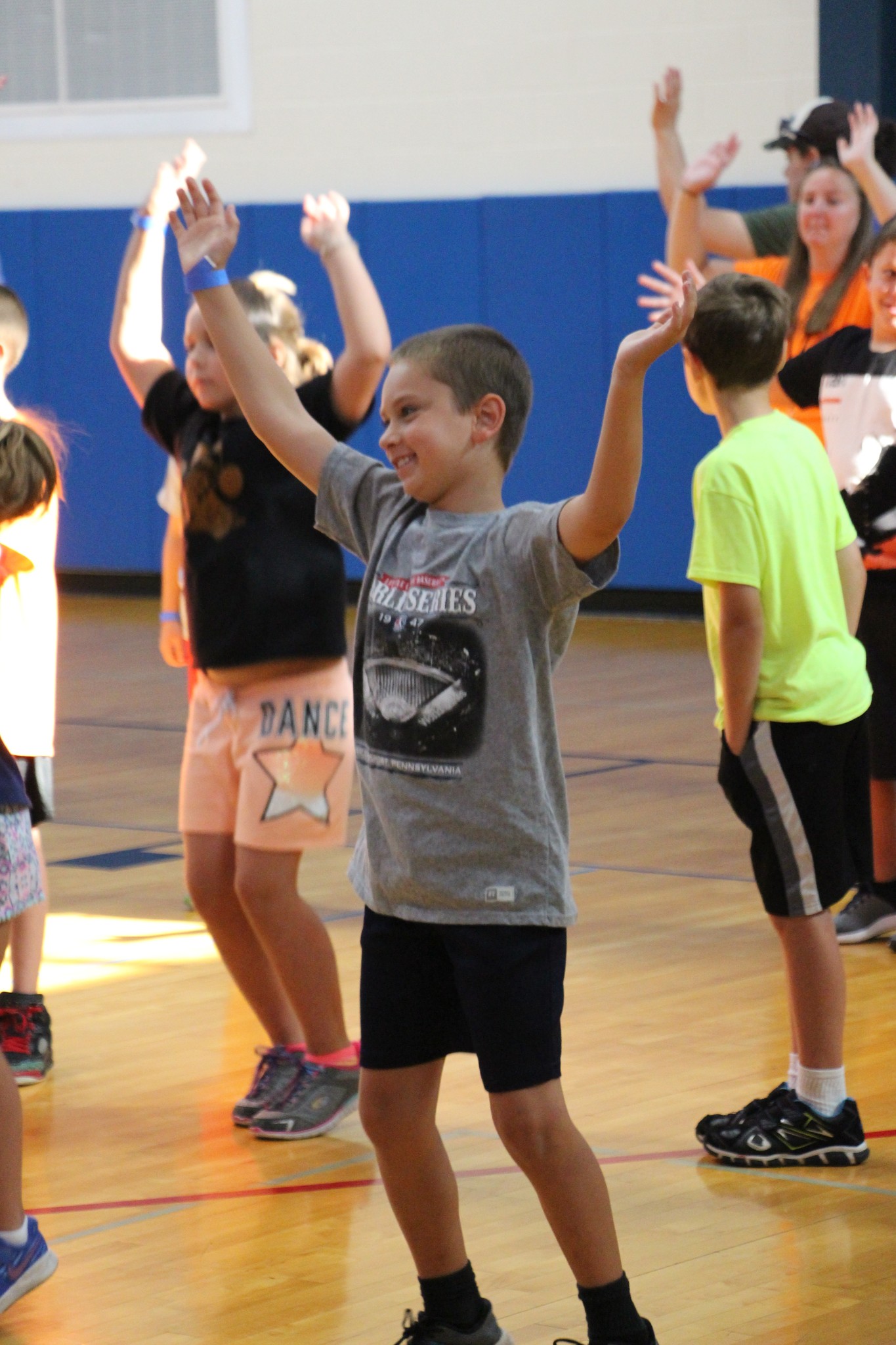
[764,96,850,151]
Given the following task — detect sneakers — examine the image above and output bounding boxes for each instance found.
[393,1298,514,1345]
[250,1040,360,1139]
[695,1082,791,1143]
[0,991,54,1087]
[232,1042,306,1125]
[703,1089,869,1168]
[834,882,896,945]
[0,1214,58,1314]
[553,1316,659,1345]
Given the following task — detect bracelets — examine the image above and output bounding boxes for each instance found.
[129,210,168,237]
[183,254,229,293]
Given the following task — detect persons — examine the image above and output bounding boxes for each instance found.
[681,273,873,1168]
[107,135,393,1141]
[169,176,698,1345]
[0,280,69,1314]
[650,68,896,944]
